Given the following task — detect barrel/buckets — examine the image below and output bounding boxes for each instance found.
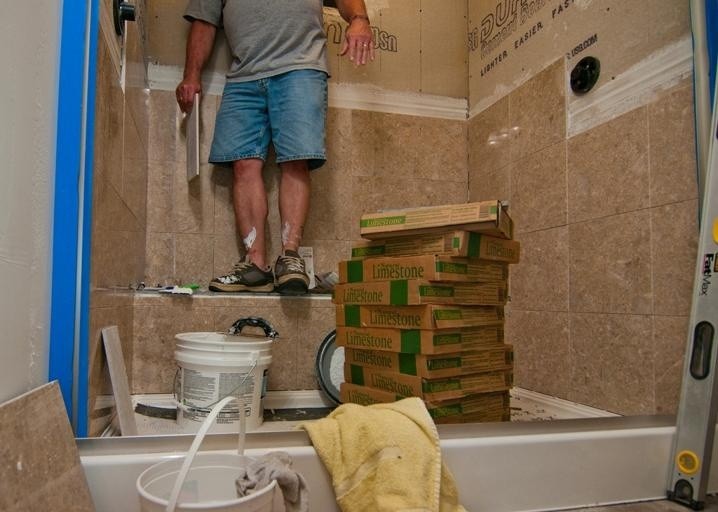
[171,331,275,431]
[138,395,277,512]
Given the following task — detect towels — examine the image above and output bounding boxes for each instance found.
[294,396,468,512]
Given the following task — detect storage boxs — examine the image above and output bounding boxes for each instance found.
[344,362,514,400]
[340,383,511,425]
[361,199,514,240]
[344,345,514,380]
[351,230,520,263]
[336,303,504,331]
[336,326,504,354]
[333,280,508,307]
[338,255,510,284]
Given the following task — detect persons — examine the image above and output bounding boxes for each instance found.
[174,0,379,294]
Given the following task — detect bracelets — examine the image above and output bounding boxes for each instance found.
[348,15,370,25]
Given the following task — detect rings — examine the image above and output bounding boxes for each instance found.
[362,43,368,48]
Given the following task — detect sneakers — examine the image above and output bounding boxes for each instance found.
[207,254,276,292]
[273,249,310,292]
